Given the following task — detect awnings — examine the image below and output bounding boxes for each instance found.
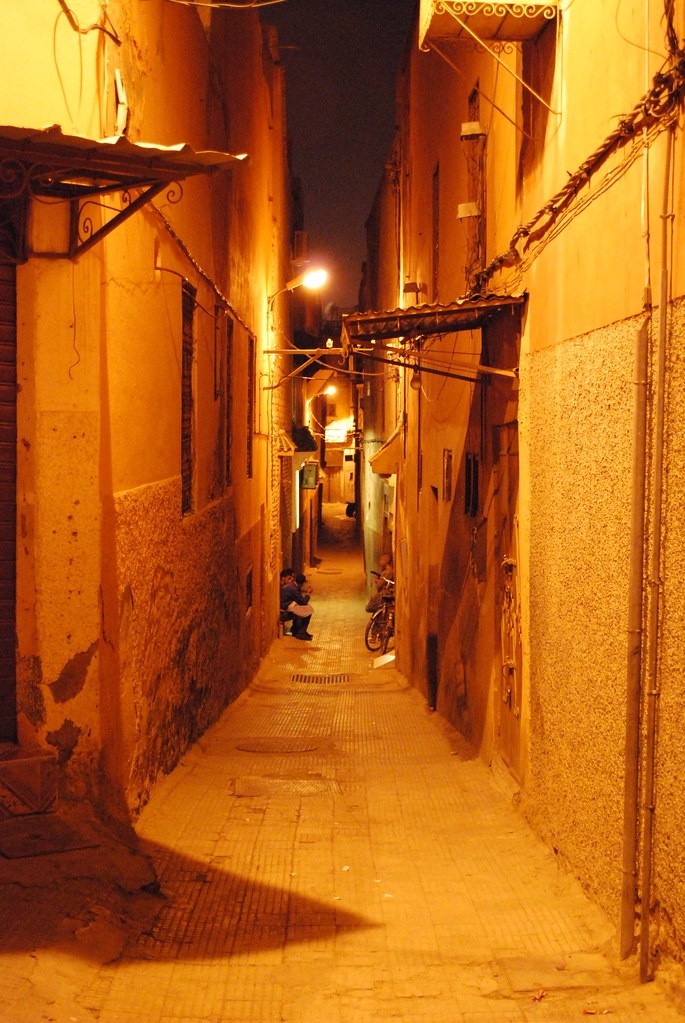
[369,431,402,475]
[419,1,561,138]
[340,293,523,382]
[0,123,249,257]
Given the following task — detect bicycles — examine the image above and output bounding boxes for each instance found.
[365,570,396,656]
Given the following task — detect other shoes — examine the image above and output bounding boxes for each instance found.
[367,637,376,643]
[290,626,312,640]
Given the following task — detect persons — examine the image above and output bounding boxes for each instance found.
[368,554,393,643]
[280,568,314,640]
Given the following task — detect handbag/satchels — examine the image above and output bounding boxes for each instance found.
[366,593,383,612]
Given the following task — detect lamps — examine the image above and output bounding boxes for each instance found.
[461,121,486,141]
[457,202,482,219]
[267,267,326,311]
[403,282,421,293]
